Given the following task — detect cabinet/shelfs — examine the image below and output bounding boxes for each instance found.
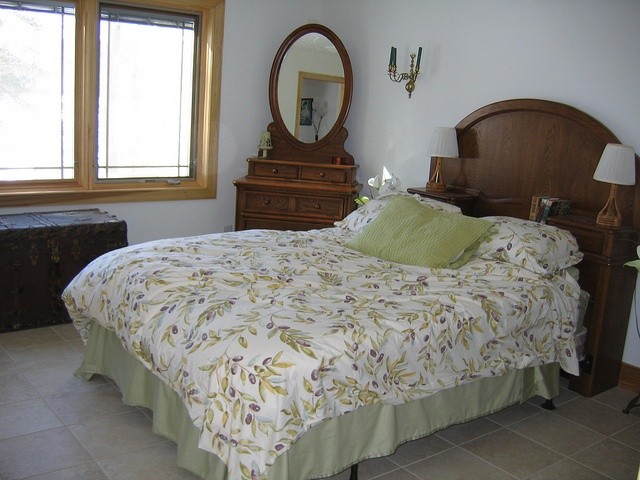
[232,158,365,231]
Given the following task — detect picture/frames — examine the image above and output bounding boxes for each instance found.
[299,99,312,125]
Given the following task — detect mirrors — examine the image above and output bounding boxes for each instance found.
[268,23,354,151]
[294,70,345,144]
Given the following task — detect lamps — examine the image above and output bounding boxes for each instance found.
[593,143,635,228]
[426,127,460,191]
[388,46,423,98]
[257,131,274,158]
[61,99,640,479]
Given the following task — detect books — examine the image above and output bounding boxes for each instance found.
[528,194,572,225]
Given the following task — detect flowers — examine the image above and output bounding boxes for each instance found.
[353,165,401,205]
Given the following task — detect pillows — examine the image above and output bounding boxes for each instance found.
[472,214,585,277]
[341,193,495,270]
[334,184,463,236]
